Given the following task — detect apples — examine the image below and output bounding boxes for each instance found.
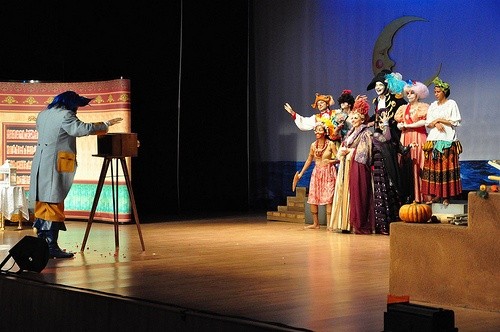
[490,185,499,192]
[479,185,489,191]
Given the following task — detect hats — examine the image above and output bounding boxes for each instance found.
[310,94,331,109]
[49,91,93,109]
[367,69,393,91]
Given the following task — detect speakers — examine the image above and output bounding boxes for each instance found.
[9,235,49,272]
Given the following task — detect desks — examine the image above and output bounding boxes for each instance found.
[0,184,29,231]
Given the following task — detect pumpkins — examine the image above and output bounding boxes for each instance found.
[399,200,434,222]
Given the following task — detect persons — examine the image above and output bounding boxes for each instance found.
[284,70,431,235]
[421,77,464,206]
[30,91,124,258]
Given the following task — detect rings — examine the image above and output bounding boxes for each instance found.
[435,122,436,124]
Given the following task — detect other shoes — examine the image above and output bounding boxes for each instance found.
[45,245,74,259]
